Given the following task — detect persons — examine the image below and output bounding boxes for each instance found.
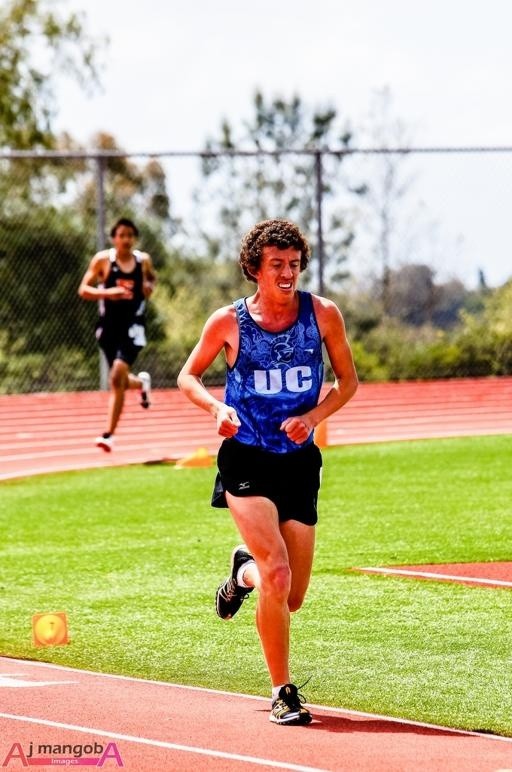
[73,214,158,453]
[170,218,363,729]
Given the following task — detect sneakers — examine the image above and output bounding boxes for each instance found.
[97,435,112,452]
[270,684,313,724]
[215,545,254,620]
[138,372,152,410]
